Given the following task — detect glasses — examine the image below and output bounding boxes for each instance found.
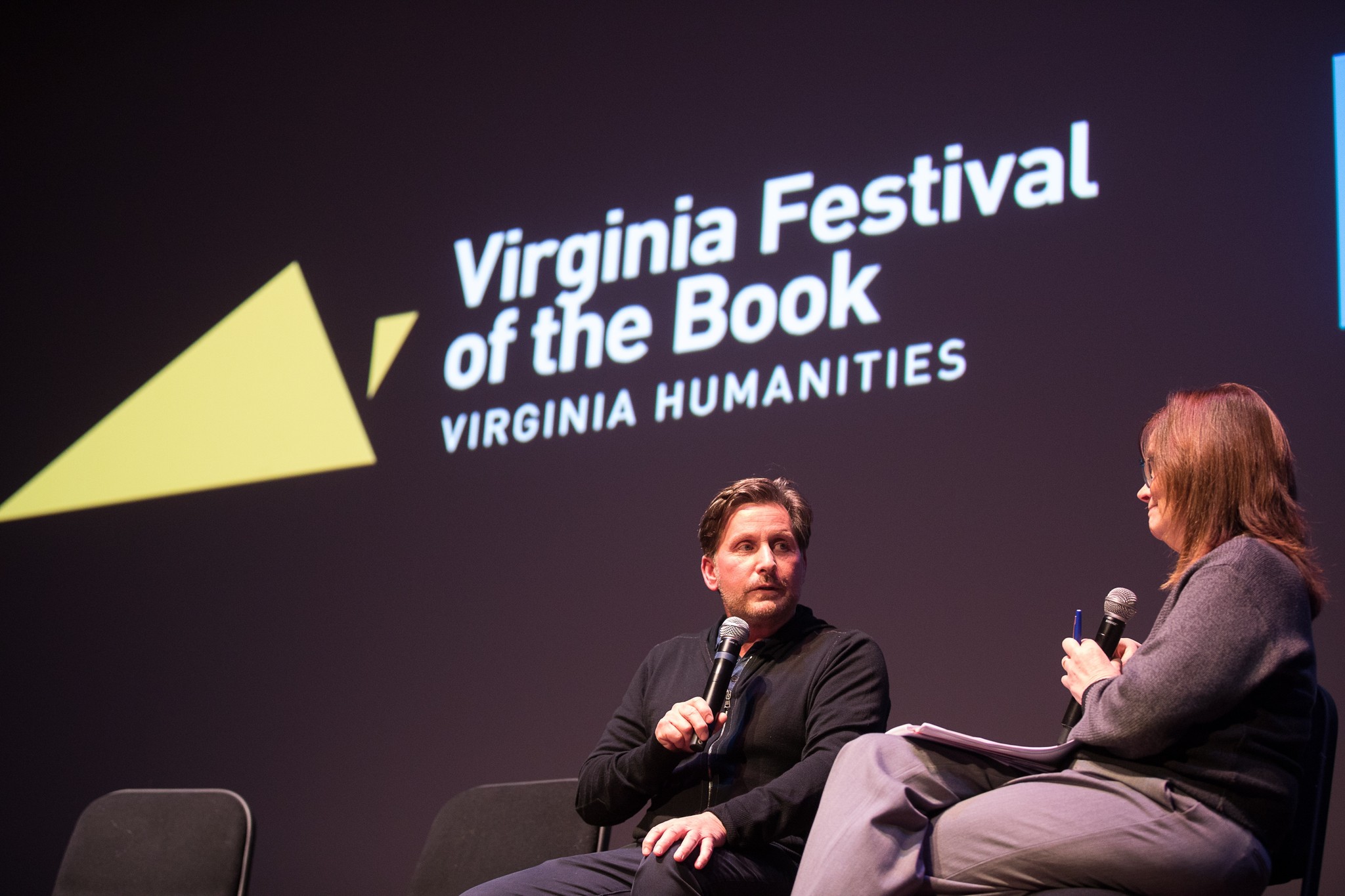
[1140,456,1155,488]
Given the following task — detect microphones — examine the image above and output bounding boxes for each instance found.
[1058,588,1137,747]
[688,616,749,753]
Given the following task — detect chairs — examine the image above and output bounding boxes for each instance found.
[408,777,612,896]
[51,788,253,895]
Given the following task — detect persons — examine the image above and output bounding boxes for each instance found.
[459,476,890,896]
[786,383,1326,896]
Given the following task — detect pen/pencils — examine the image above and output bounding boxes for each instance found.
[1073,609,1082,644]
